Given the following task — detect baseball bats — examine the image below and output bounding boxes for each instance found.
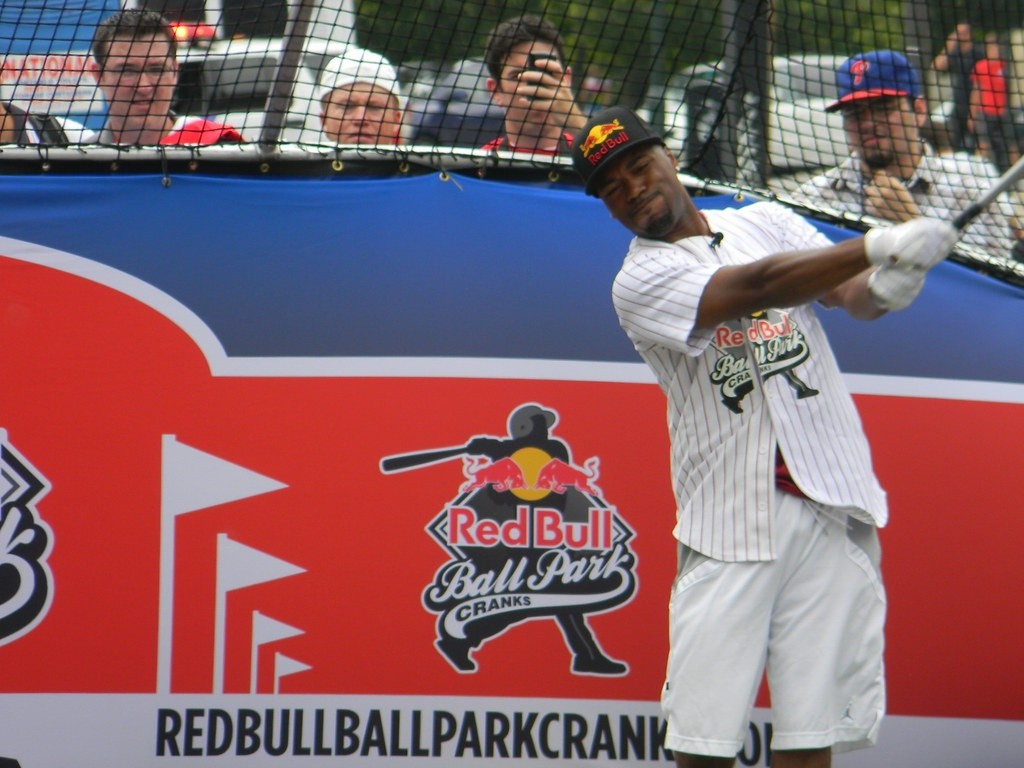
[867,153,1024,307]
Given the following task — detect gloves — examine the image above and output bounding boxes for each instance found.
[869,263,924,311]
[865,218,960,267]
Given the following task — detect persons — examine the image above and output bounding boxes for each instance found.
[0,98,94,148]
[82,9,244,145]
[481,14,589,155]
[932,21,1013,175]
[319,49,402,144]
[786,48,1014,259]
[569,103,958,768]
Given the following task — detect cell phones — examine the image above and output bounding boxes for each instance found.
[526,52,557,101]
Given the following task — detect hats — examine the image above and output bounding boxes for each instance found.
[568,104,662,192]
[318,49,400,108]
[825,48,920,116]
